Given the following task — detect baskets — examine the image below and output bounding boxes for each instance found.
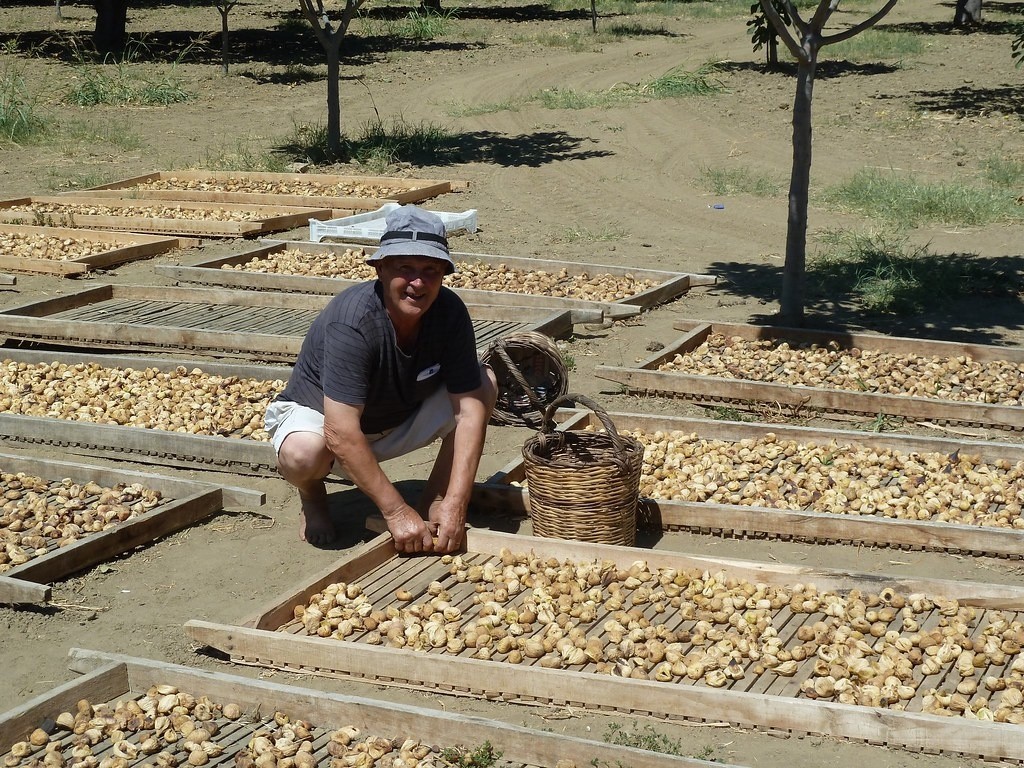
[479,331,570,427]
[522,394,644,548]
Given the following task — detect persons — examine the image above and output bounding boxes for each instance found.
[263,204,499,554]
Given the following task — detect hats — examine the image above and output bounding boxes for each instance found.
[365,204,454,275]
